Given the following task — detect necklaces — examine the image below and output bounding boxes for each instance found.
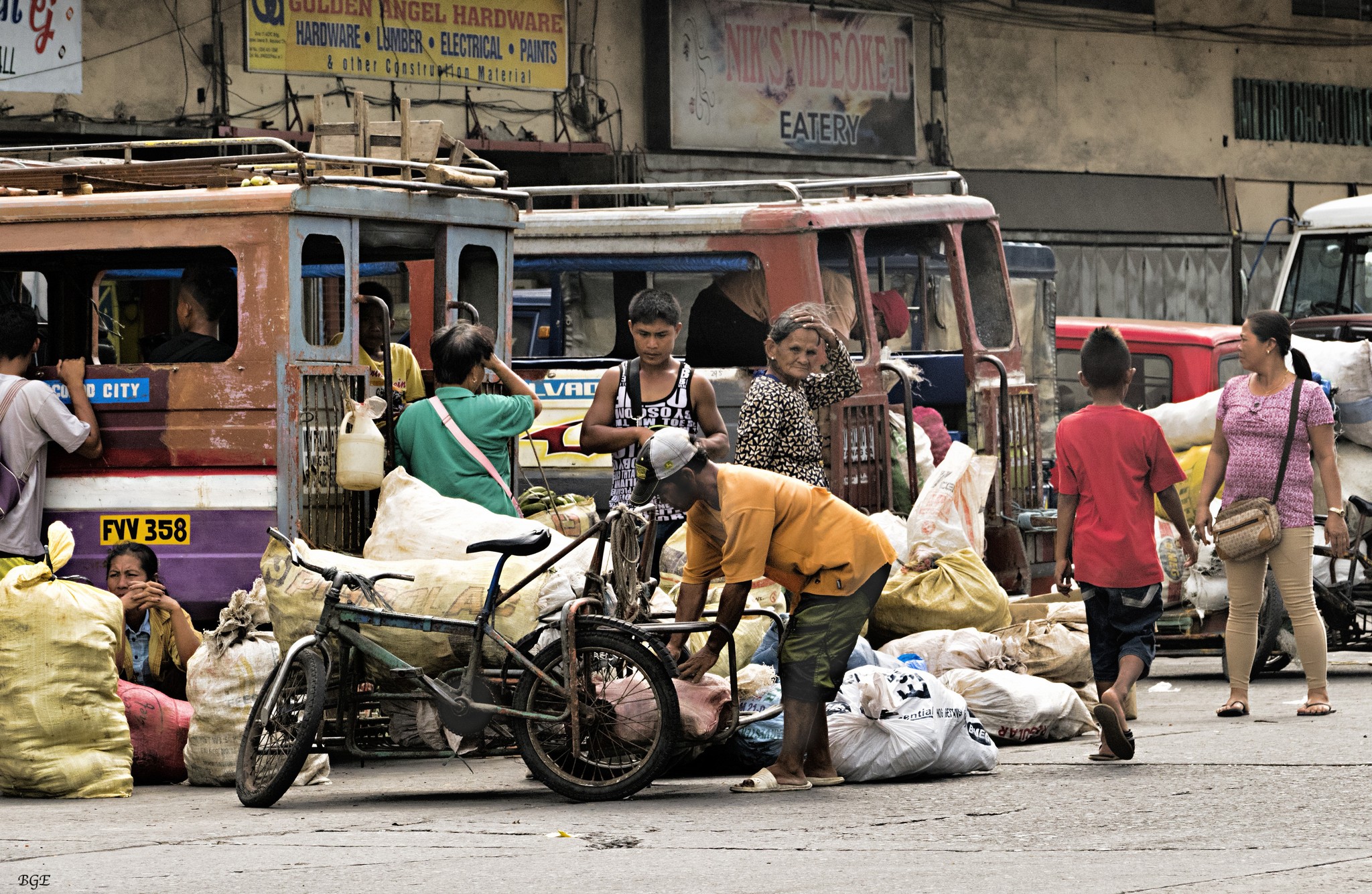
[1257,370,1288,396]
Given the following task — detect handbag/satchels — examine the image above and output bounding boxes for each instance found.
[0,379,45,520]
[1212,497,1281,563]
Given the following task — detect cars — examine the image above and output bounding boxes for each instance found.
[1053,313,1253,424]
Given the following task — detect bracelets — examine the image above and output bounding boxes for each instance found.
[1328,507,1344,517]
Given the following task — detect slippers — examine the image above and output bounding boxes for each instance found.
[806,776,845,785]
[729,767,812,792]
[1217,701,1250,717]
[1297,703,1337,716]
[1093,704,1135,760]
[1088,744,1120,761]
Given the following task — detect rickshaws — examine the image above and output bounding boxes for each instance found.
[1221,494,1372,685]
[233,521,794,811]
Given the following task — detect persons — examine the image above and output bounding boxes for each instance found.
[0,303,101,572]
[143,259,236,363]
[106,541,203,700]
[1049,326,1197,761]
[395,320,543,518]
[329,281,425,475]
[686,264,910,368]
[580,287,730,587]
[1194,310,1350,718]
[629,426,897,791]
[733,301,864,614]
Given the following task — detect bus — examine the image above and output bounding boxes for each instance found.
[513,169,1060,593]
[0,132,534,630]
[1237,192,1372,349]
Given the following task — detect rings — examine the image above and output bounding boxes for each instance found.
[811,316,814,322]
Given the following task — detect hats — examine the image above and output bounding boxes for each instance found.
[870,289,910,339]
[629,427,698,507]
